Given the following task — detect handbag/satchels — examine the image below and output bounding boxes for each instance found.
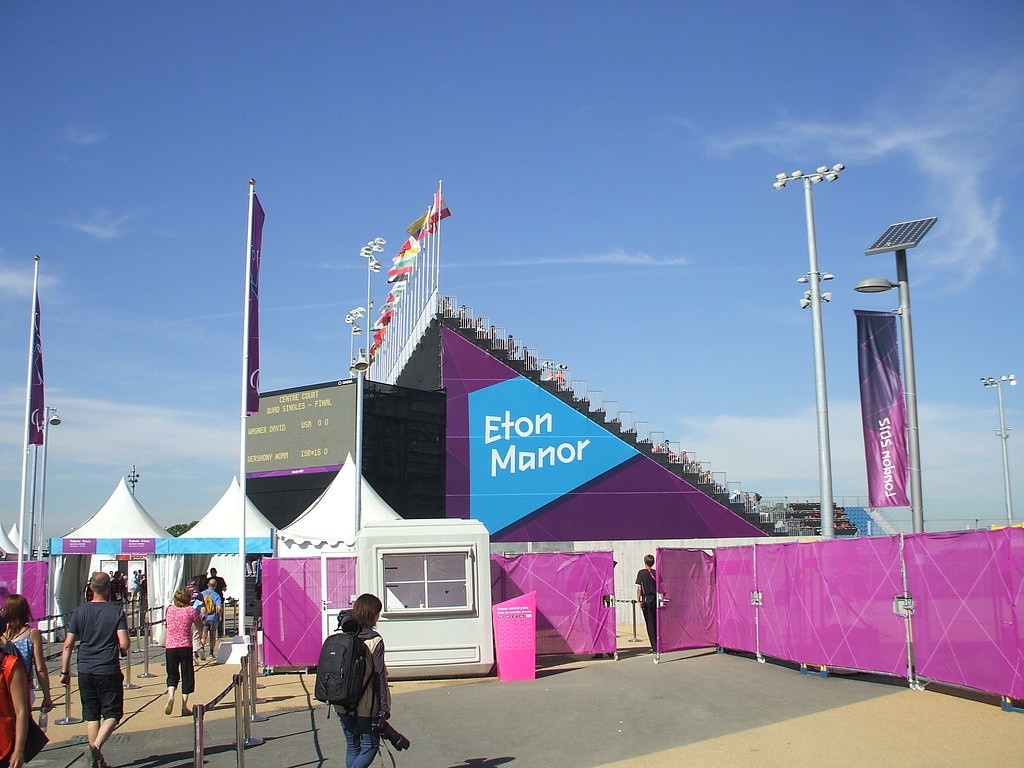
[12,717,48,763]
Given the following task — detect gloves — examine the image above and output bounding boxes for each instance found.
[375,710,390,723]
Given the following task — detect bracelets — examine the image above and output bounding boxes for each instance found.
[61,671,69,674]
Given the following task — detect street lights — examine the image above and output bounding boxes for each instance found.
[346,237,389,536]
[980,375,1017,525]
[772,163,846,537]
[854,217,937,530]
[38,405,61,563]
[127,465,140,496]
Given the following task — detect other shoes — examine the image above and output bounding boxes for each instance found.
[608,652,619,658]
[164,696,174,715]
[209,655,216,660]
[97,754,107,768]
[182,708,191,716]
[85,744,101,768]
[193,653,199,666]
[200,649,206,660]
[591,653,603,659]
[648,649,657,654]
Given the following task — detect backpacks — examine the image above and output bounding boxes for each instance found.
[314,634,375,711]
[0,626,30,663]
[201,591,215,615]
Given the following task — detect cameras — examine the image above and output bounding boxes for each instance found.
[371,710,411,751]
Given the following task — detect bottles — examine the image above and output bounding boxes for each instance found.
[39,708,48,733]
[120,649,127,657]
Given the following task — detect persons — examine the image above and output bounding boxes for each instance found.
[635,554,666,654]
[60,572,130,768]
[443,297,539,369]
[165,586,204,716]
[245,554,261,577]
[657,439,714,483]
[86,569,147,606]
[333,593,392,768]
[0,594,54,713]
[0,615,32,768]
[186,567,227,666]
[252,570,262,630]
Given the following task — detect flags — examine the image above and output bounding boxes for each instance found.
[366,185,452,365]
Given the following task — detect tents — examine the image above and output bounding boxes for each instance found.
[0,523,30,554]
[278,452,405,609]
[168,476,279,605]
[50,475,176,647]
